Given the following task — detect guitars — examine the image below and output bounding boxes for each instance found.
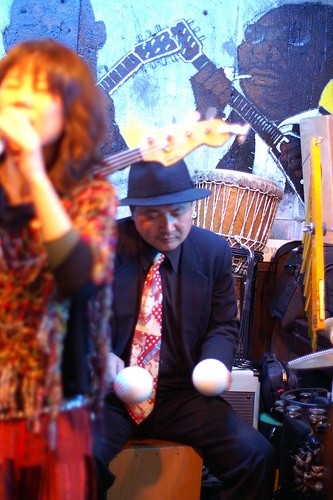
[96,120,229,178]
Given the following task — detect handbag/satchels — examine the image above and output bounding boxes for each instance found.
[262,350,300,409]
[268,242,333,335]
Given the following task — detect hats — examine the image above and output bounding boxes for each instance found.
[116,159,212,206]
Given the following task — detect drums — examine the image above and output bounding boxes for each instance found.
[190,170,282,252]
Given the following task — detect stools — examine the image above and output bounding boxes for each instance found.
[106,437,204,500]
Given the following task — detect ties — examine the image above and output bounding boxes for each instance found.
[125,254,164,424]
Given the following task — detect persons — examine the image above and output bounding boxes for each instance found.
[0,37,119,499]
[87,157,277,499]
